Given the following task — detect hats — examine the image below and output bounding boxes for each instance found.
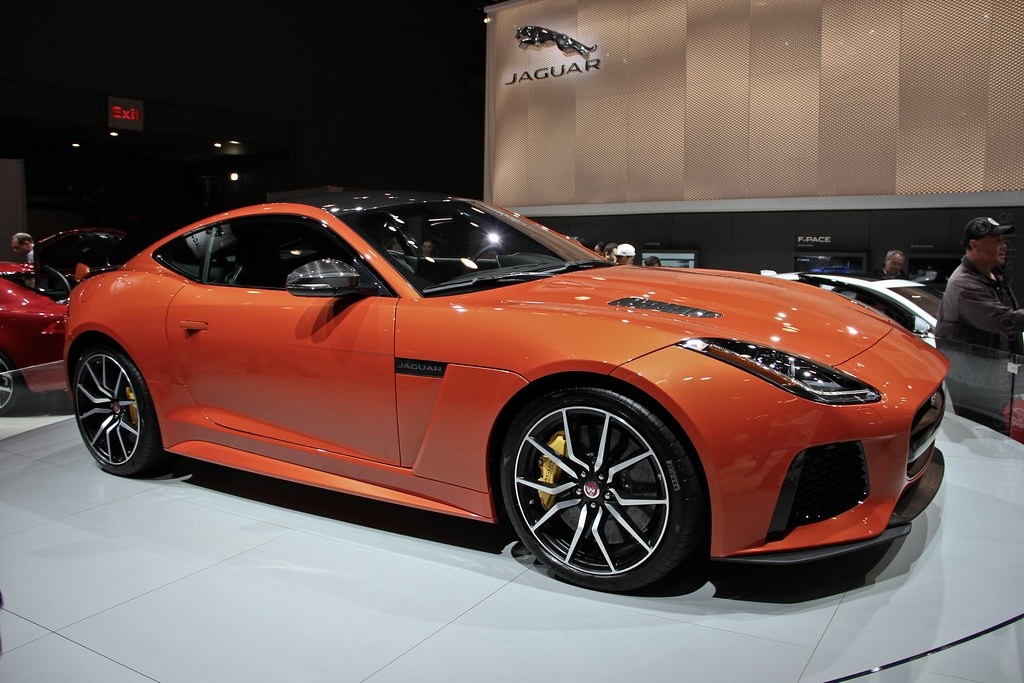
[963,216,1016,241]
[613,244,636,256]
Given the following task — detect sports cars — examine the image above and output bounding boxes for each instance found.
[61,190,952,597]
[756,266,1024,442]
[0,227,129,422]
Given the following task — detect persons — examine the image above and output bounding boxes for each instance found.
[11,233,35,287]
[388,237,662,267]
[934,217,1024,433]
[871,251,908,279]
